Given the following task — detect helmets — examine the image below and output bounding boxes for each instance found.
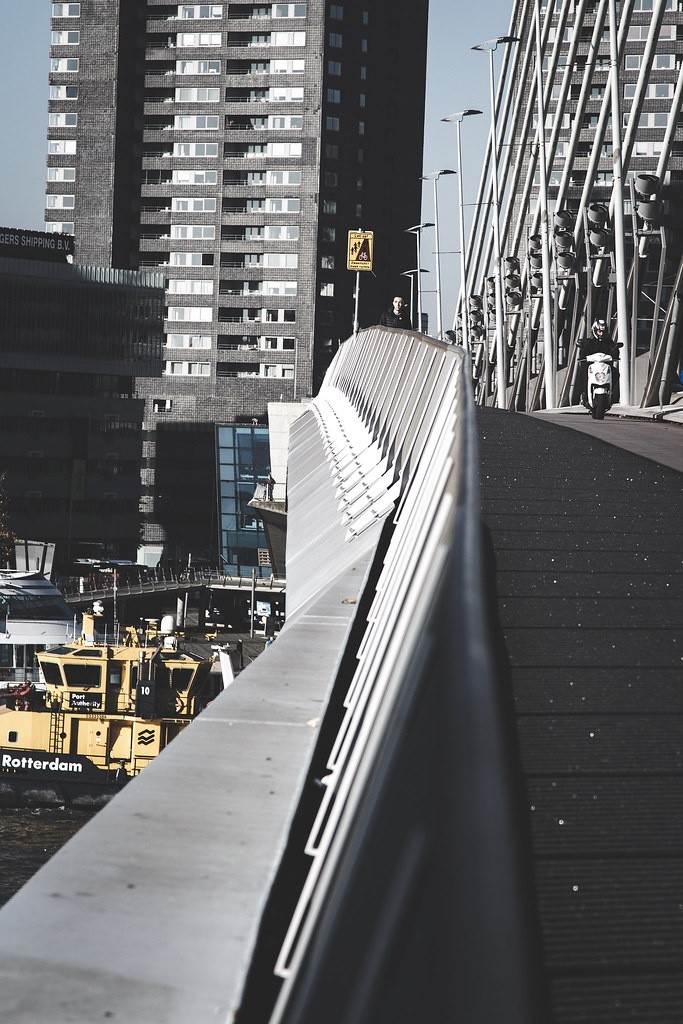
[591,320,608,342]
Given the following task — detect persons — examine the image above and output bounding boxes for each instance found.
[442,330,456,345]
[580,320,620,404]
[237,638,244,669]
[380,296,410,329]
[266,474,276,501]
[265,636,274,649]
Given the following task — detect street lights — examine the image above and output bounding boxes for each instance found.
[418,168,459,344]
[469,34,525,411]
[438,108,484,350]
[404,222,436,334]
[400,268,431,330]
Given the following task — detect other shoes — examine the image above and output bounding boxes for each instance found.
[582,394,588,400]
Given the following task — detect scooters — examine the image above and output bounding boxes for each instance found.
[576,337,624,420]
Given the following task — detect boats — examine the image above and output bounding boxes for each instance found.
[0,570,249,808]
